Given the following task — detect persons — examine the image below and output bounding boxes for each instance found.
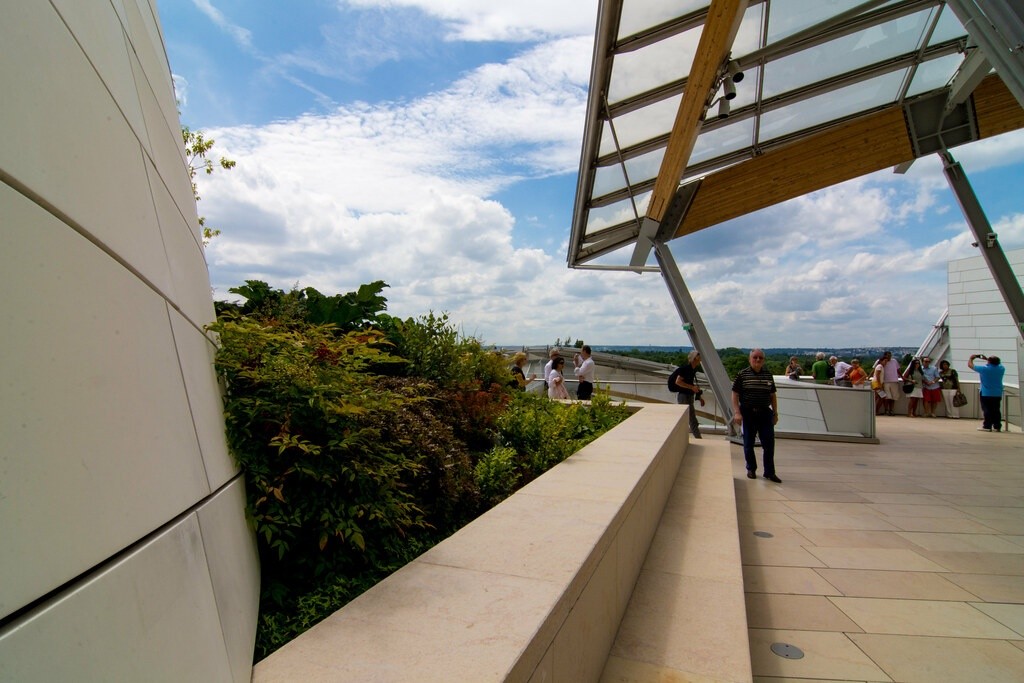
[868,351,905,416]
[732,349,782,483]
[509,352,537,392]
[828,356,852,386]
[574,345,595,400]
[850,359,867,388]
[871,357,888,415]
[903,360,929,417]
[968,354,1005,432]
[812,352,830,384]
[675,352,705,439]
[785,356,804,380]
[939,360,962,419]
[921,357,942,418]
[544,347,571,399]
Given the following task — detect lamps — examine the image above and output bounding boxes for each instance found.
[723,60,744,83]
[717,77,736,101]
[709,96,730,119]
[986,233,997,247]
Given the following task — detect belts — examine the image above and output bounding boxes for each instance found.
[750,408,764,412]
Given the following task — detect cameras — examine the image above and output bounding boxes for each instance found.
[695,389,702,400]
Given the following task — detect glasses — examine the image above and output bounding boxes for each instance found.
[753,356,763,360]
[558,361,564,365]
[925,360,930,363]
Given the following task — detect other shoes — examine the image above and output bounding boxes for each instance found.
[747,470,756,478]
[891,409,895,415]
[931,413,936,417]
[994,429,1000,432]
[977,426,991,431]
[885,409,889,416]
[921,413,928,417]
[763,474,782,483]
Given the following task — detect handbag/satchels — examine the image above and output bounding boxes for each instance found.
[872,379,878,389]
[953,391,968,407]
[902,383,915,394]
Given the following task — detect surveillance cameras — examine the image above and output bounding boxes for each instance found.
[987,232,998,240]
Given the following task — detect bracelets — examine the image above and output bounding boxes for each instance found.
[970,358,973,360]
[932,380,935,383]
[773,411,779,416]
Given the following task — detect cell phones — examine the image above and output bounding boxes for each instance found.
[975,355,980,358]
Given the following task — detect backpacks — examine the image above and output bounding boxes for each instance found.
[667,368,688,392]
